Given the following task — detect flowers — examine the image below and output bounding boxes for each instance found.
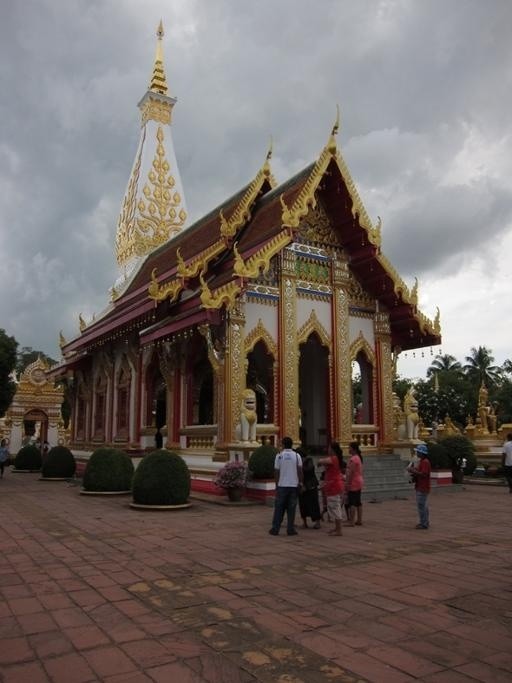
[213,462,249,489]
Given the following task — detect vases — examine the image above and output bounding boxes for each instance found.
[228,487,244,500]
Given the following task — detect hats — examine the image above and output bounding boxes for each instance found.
[413,444,427,454]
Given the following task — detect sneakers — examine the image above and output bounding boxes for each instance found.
[269,528,277,535]
[288,531,298,535]
[301,523,308,529]
[312,524,321,528]
[415,523,428,528]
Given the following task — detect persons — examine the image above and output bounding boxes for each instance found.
[318,470,334,523]
[0,436,12,476]
[340,441,365,525]
[314,441,344,537]
[292,446,321,529]
[500,431,512,495]
[268,436,305,536]
[332,460,354,520]
[405,443,432,529]
